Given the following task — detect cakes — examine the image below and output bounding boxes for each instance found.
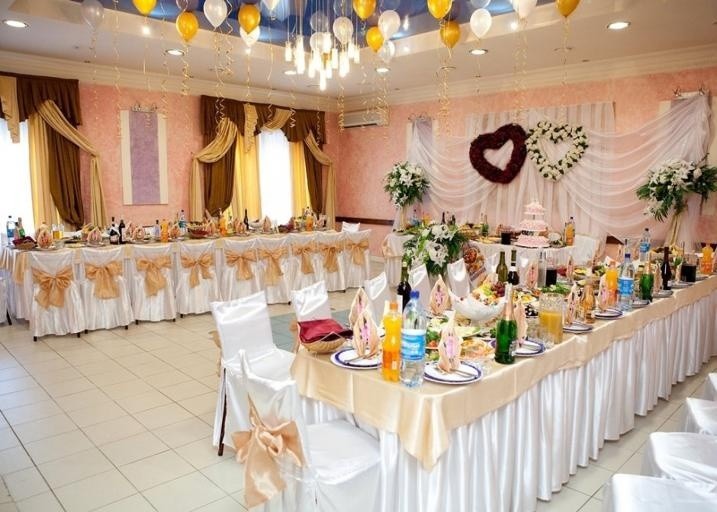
[516,233,550,246]
[520,219,546,232]
[525,201,545,214]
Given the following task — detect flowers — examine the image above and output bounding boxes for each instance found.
[380,160,431,209]
[634,157,717,222]
[467,118,588,183]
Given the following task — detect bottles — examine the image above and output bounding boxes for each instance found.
[408,204,577,246]
[381,228,717,386]
[6,206,322,251]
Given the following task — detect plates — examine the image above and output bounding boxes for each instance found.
[421,267,716,384]
[332,347,387,369]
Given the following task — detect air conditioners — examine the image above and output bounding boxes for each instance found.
[338,108,390,128]
